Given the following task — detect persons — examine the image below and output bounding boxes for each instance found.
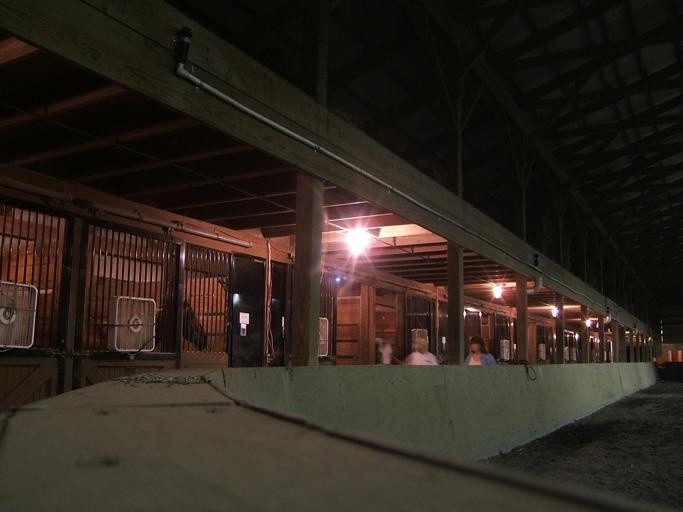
[464,337,496,365]
[404,339,437,365]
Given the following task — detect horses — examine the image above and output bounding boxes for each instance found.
[82,300,209,352]
